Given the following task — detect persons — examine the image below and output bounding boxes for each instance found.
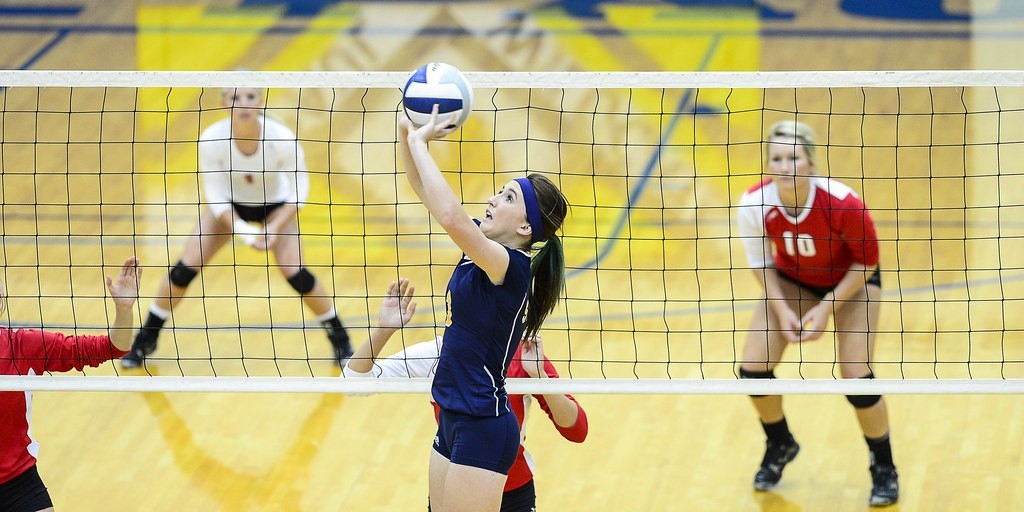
[337,276,590,511]
[2,256,145,512]
[388,102,570,512]
[119,70,356,372]
[737,119,902,509]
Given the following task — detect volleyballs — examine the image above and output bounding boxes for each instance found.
[402,63,474,130]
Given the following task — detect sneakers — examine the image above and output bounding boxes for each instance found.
[753,433,800,491]
[868,462,898,507]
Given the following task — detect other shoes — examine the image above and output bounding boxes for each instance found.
[329,332,354,367]
[122,333,157,369]
